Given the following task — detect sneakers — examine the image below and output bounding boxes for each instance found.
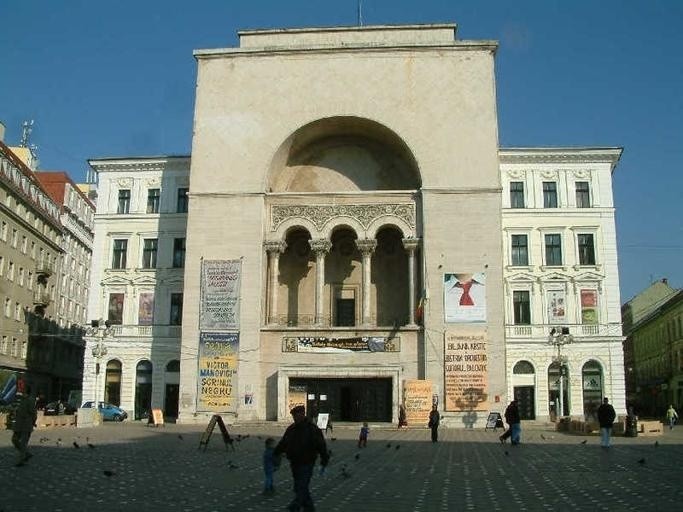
[14,453,34,469]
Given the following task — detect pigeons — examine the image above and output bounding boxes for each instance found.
[56,438,64,450]
[550,327,556,336]
[227,460,239,470]
[637,459,646,464]
[342,468,352,478]
[73,442,79,449]
[103,470,115,477]
[655,441,659,448]
[86,437,94,448]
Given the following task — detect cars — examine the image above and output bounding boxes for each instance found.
[80,399,127,422]
[43,401,76,416]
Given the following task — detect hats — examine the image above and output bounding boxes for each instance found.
[291,405,305,413]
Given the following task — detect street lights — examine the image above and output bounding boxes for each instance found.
[85,317,111,409]
[549,327,572,416]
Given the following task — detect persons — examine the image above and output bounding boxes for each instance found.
[397,405,406,429]
[665,403,678,429]
[109,297,116,320]
[498,401,513,444]
[428,404,440,444]
[5,384,37,468]
[356,421,369,447]
[508,400,521,444]
[114,301,122,323]
[444,272,486,323]
[596,397,616,449]
[262,437,280,496]
[271,404,329,511]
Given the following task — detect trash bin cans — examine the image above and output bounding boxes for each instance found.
[625,415,637,437]
